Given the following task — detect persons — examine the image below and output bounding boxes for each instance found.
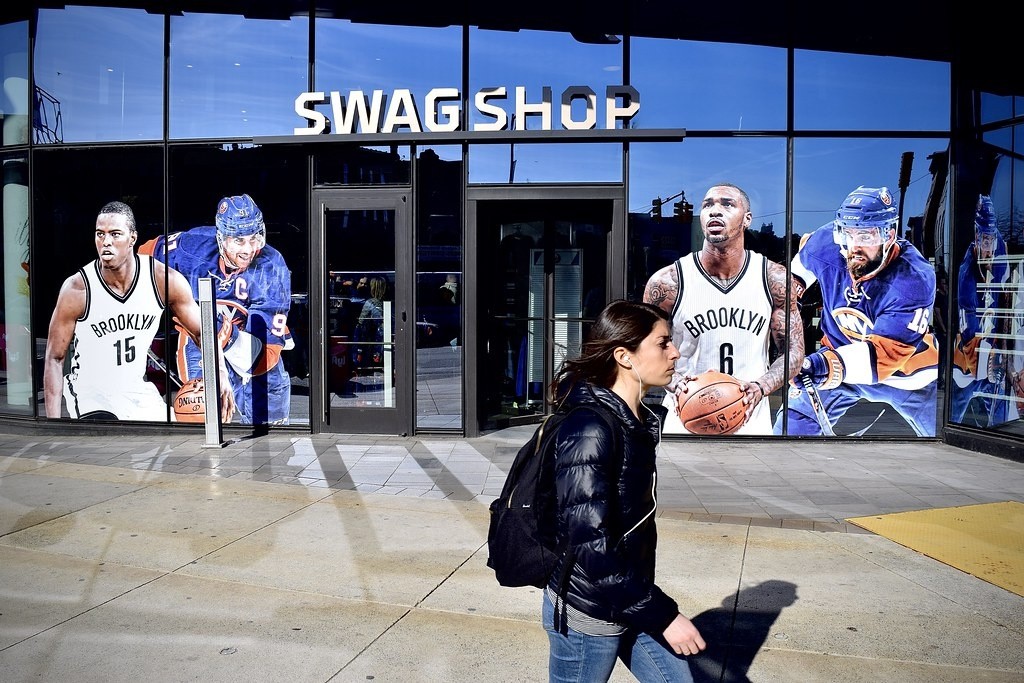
[949,195,1012,426]
[771,185,938,438]
[43,201,236,422]
[440,273,460,304]
[541,300,706,683]
[329,271,390,364]
[642,184,804,435]
[137,193,295,426]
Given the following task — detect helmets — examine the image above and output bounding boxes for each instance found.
[973,194,999,244]
[216,194,265,238]
[833,184,900,239]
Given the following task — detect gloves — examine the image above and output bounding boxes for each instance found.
[788,347,847,393]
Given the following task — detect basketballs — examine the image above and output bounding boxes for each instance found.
[677,371,748,437]
[173,378,234,425]
[1014,370,1024,411]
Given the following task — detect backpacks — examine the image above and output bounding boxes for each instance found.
[484,402,627,589]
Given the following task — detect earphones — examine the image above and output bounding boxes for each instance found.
[624,356,629,362]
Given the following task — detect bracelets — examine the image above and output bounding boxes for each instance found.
[754,381,764,396]
[219,367,228,373]
